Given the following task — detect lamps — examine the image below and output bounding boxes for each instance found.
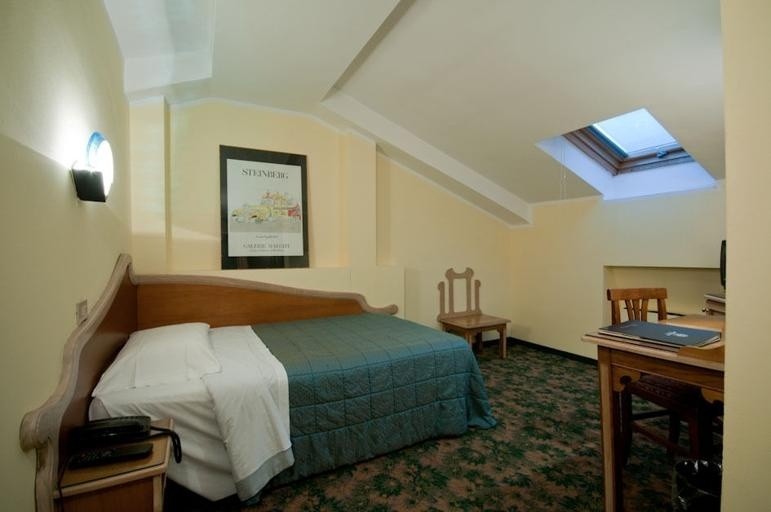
[71,130,117,203]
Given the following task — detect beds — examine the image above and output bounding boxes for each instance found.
[93,315,496,497]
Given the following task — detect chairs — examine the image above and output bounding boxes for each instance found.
[437,267,509,360]
[607,287,723,465]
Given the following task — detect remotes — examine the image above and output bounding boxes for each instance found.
[68,443,153,470]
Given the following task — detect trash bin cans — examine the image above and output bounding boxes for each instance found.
[671,460,722,512]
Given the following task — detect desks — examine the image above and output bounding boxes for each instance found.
[580,314,726,511]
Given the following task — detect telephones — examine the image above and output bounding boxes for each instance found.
[68,416,150,452]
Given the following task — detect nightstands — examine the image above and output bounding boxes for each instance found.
[54,416,175,511]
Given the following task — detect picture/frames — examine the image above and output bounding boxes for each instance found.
[218,143,312,273]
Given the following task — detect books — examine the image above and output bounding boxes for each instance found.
[584,330,721,354]
[598,317,724,348]
